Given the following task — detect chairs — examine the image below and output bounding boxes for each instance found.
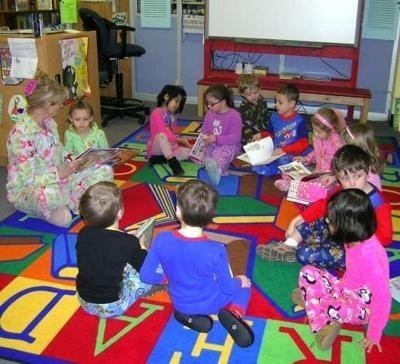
[77,7,150,126]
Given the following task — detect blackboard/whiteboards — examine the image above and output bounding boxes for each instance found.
[205,0,365,48]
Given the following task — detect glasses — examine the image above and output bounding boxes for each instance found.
[204,96,227,106]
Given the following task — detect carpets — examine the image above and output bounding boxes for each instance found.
[1,118,400,363]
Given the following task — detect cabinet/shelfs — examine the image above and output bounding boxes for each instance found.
[203,34,358,122]
[1,28,101,165]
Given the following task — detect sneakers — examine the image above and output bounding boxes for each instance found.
[149,154,168,165]
[172,311,215,333]
[167,156,184,175]
[256,240,298,263]
[291,286,306,308]
[217,308,255,349]
[316,321,343,351]
[204,157,221,184]
[274,178,290,191]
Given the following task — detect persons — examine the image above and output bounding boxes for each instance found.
[139,179,254,347]
[148,74,311,185]
[75,181,159,320]
[6,69,115,229]
[257,106,393,355]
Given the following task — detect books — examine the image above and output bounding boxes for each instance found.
[77,147,142,169]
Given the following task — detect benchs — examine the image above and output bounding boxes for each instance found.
[196,73,370,125]
